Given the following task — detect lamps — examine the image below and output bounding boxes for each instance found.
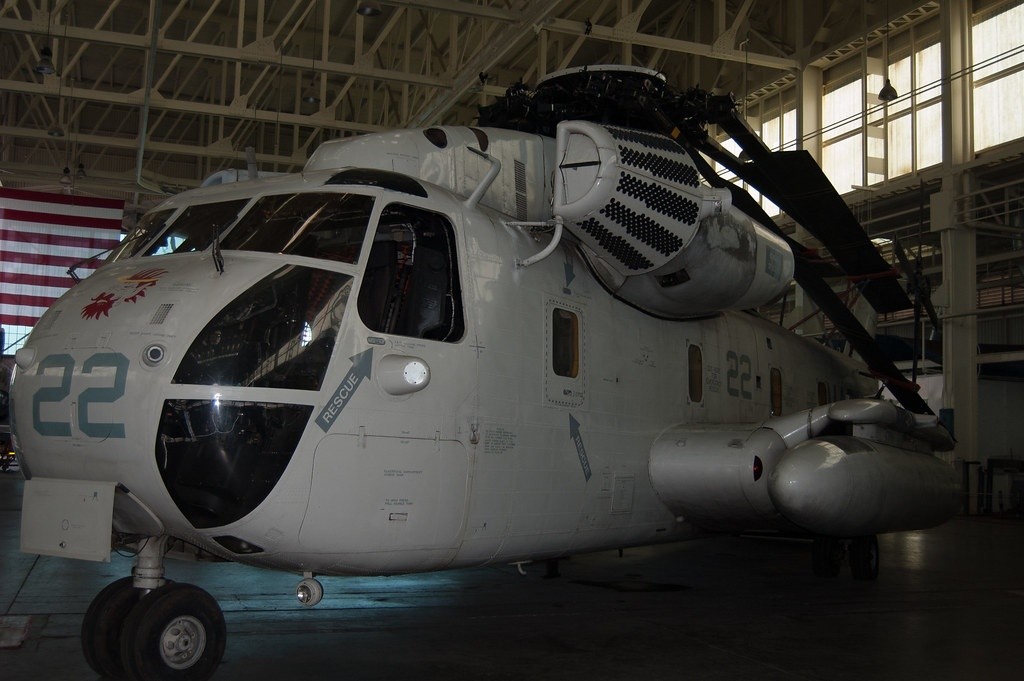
[59,80,72,185]
[302,0,321,104]
[48,20,64,137]
[75,163,86,179]
[878,0,897,101]
[32,0,56,74]
[356,0,384,17]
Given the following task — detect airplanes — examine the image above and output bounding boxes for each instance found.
[1,58,974,681]
